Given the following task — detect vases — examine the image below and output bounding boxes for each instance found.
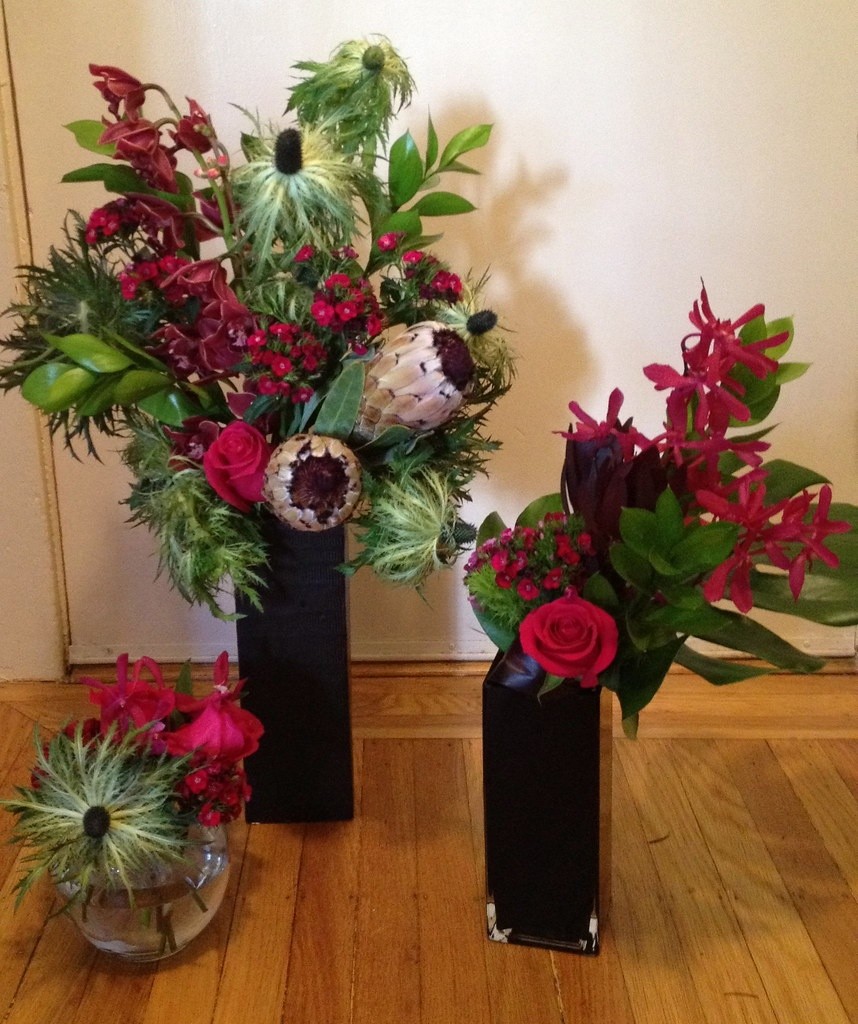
[233,503,355,826]
[66,816,229,964]
[481,651,611,954]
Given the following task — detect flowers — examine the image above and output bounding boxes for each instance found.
[461,280,858,742]
[0,36,521,627]
[0,650,266,955]
